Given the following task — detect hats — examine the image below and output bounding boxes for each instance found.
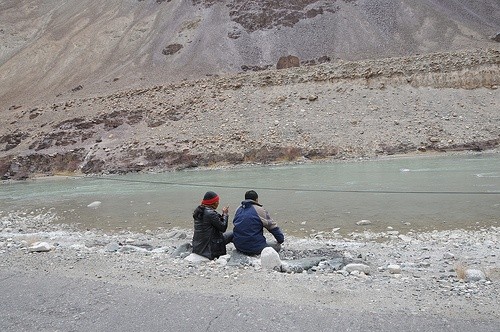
[202,191,219,204]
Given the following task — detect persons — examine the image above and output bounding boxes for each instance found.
[192,191,233,260]
[232,190,284,256]
